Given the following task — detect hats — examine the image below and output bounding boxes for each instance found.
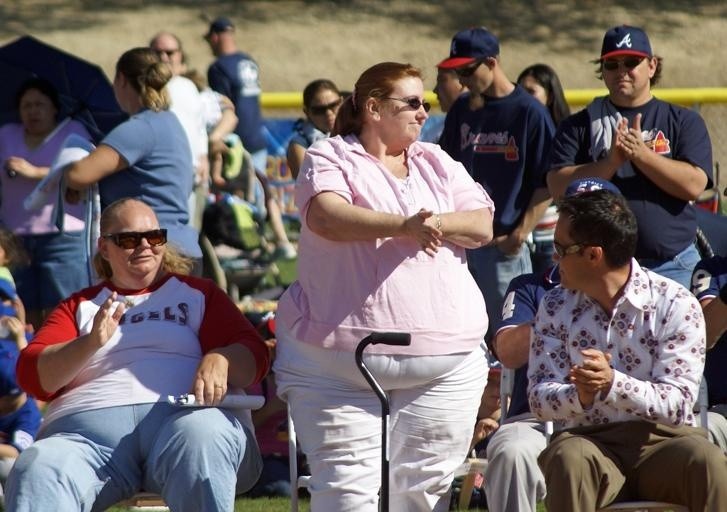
[203,17,234,39]
[438,27,498,69]
[561,176,617,196]
[598,26,650,58]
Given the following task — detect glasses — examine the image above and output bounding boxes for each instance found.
[600,59,642,71]
[386,96,432,111]
[103,228,166,250]
[310,99,339,116]
[154,47,174,54]
[453,61,485,77]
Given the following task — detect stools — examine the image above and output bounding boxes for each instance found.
[113,490,172,511]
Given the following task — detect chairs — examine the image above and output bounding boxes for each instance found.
[282,398,488,512]
[543,359,712,512]
[498,362,520,424]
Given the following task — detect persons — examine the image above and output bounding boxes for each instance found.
[0,11,296,511]
[273,59,494,510]
[420,25,711,450]
[492,177,727,512]
